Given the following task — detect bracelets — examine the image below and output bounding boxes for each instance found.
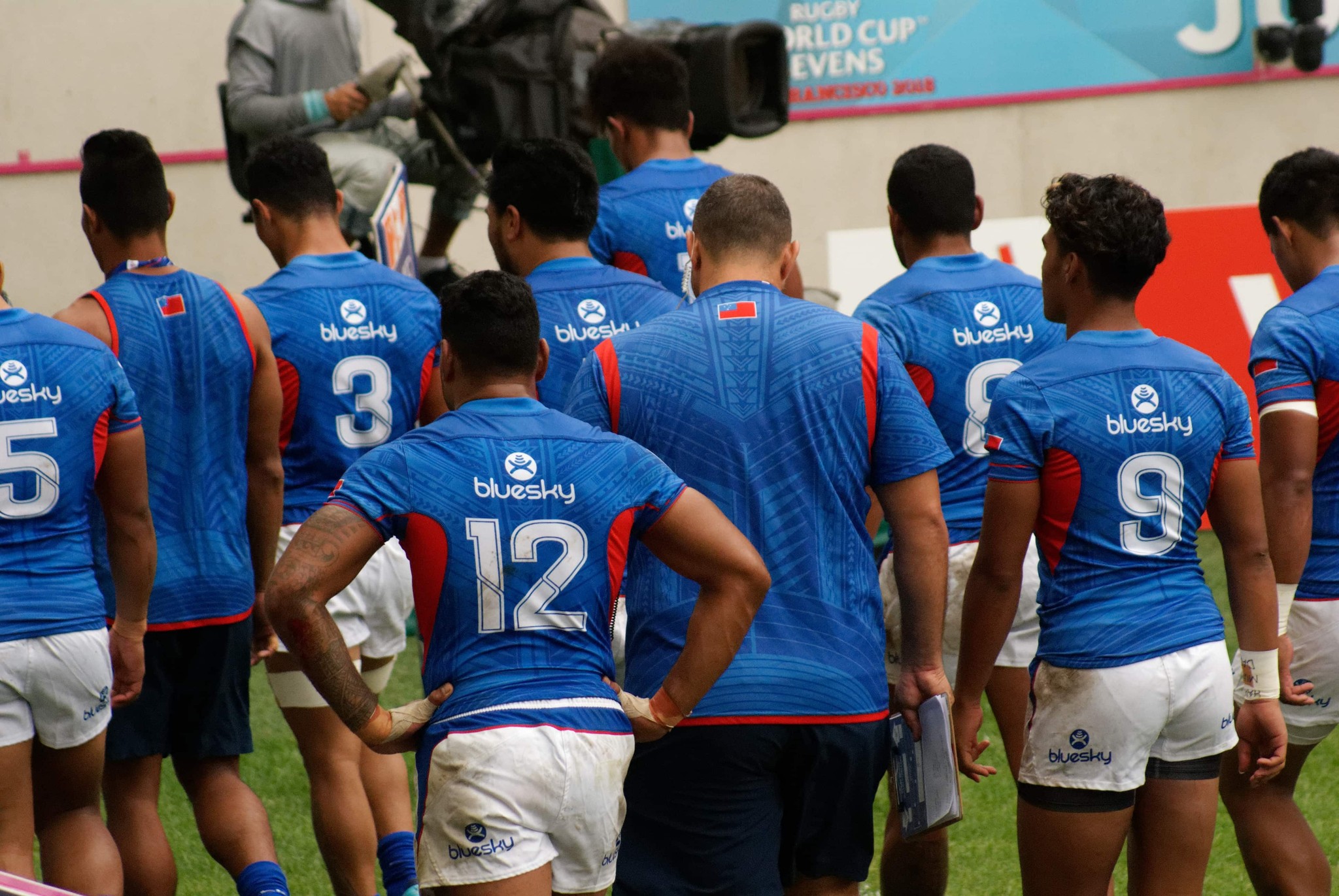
[1239,649,1280,700]
[1276,583,1298,637]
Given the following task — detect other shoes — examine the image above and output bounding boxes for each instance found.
[417,262,462,299]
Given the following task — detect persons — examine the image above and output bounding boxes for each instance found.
[1217,146,1339,896]
[266,271,772,896]
[54,128,284,896]
[0,263,157,896]
[486,134,691,414]
[225,0,482,289]
[237,137,455,896]
[847,145,1070,896]
[566,174,955,896]
[954,174,1289,896]
[588,34,806,302]
